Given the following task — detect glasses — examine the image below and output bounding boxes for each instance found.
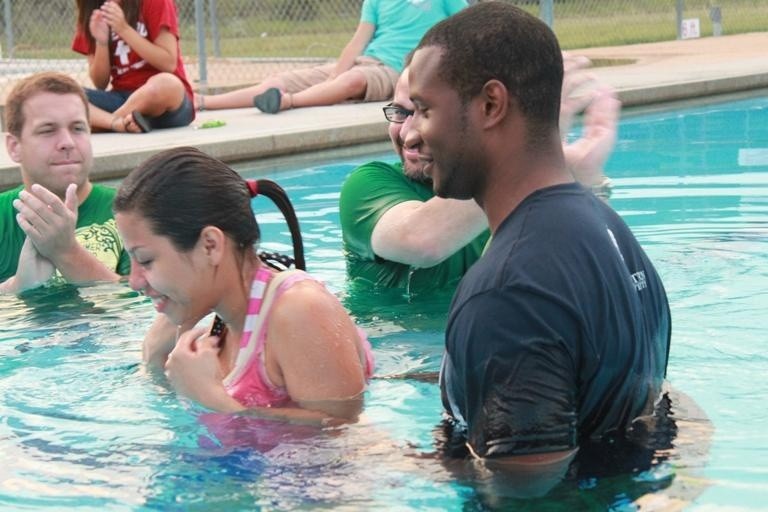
[382,97,415,125]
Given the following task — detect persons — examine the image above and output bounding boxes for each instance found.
[71,0,196,134]
[339,50,622,333]
[112,146,375,457]
[405,1,672,502]
[0,72,151,326]
[192,0,470,115]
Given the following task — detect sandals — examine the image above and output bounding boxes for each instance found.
[110,108,155,135]
[252,87,294,115]
